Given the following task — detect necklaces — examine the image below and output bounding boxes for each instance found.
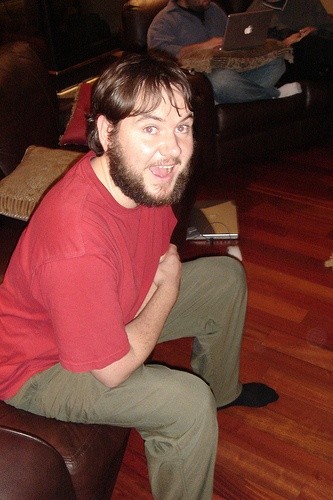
[262,0,289,12]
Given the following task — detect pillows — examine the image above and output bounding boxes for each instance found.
[0,144,87,223]
[176,38,295,74]
[58,81,95,147]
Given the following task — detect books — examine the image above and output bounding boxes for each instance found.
[182,200,239,241]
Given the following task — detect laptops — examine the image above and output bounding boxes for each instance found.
[185,199,240,239]
[218,10,271,50]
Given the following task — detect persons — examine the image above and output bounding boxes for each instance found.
[0,51,59,175]
[245,0,333,43]
[147,0,302,104]
[0,52,280,500]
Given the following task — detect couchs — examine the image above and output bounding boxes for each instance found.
[0,39,185,500]
[121,0,333,175]
